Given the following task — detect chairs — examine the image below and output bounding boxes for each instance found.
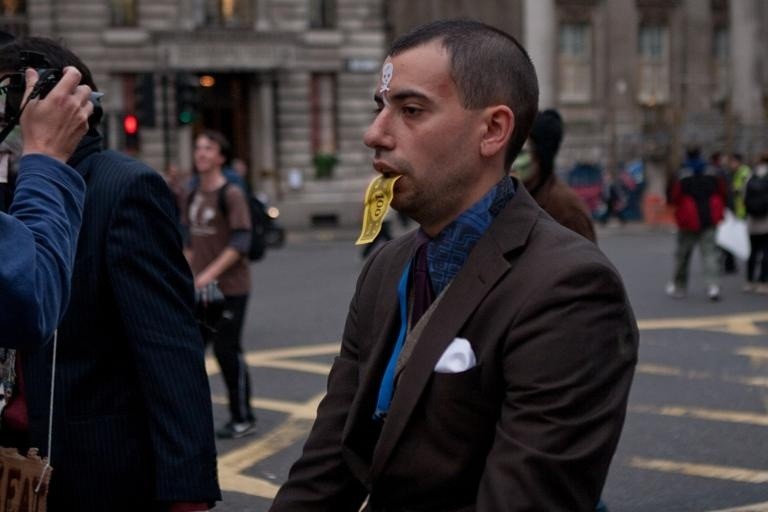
[217,421,258,438]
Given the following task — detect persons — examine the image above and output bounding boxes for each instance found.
[166,129,257,440]
[0,35,223,512]
[512,108,600,245]
[662,144,766,297]
[267,16,639,512]
[0,63,94,415]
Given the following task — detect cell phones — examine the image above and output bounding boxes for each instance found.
[3,50,103,137]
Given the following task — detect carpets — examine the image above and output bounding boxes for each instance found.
[409,244,432,327]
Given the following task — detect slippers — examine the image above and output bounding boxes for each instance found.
[188,174,281,260]
